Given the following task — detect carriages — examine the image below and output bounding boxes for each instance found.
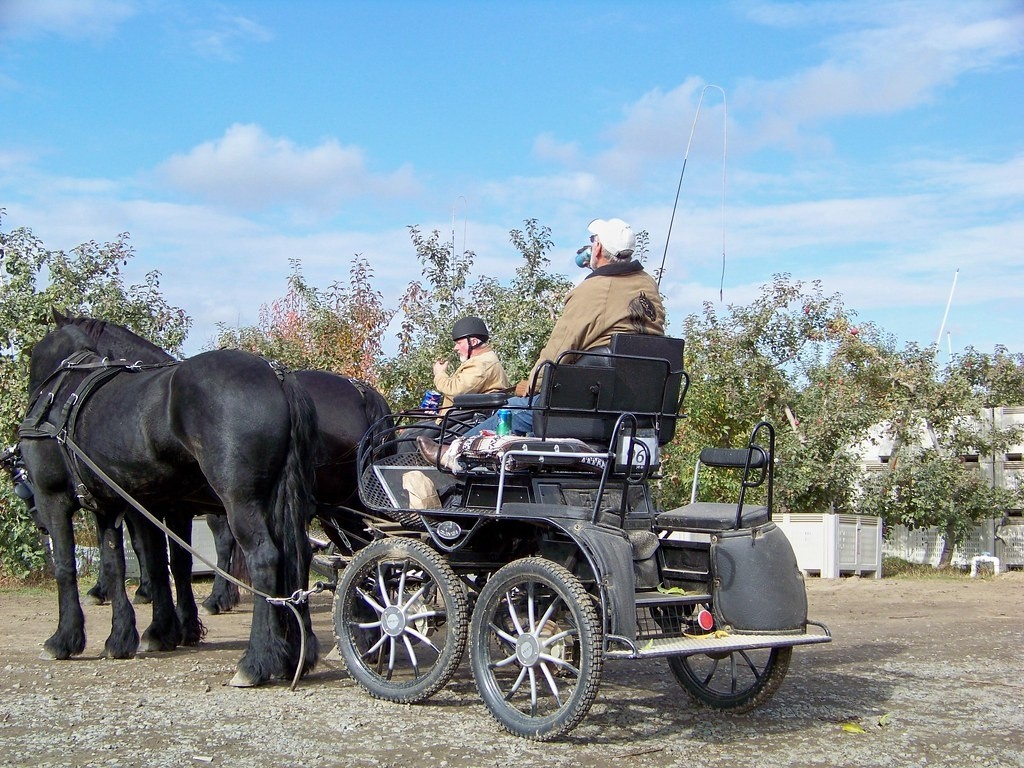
[0,306,832,742]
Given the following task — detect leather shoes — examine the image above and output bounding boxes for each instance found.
[417,435,461,470]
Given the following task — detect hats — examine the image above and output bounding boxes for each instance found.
[587,217,636,255]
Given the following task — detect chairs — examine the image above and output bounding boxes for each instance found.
[653,422,773,531]
[527,334,692,440]
[435,393,512,474]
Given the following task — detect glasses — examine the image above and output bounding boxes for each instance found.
[588,234,598,242]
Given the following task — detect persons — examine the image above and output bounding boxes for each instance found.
[397,316,510,452]
[415,218,666,472]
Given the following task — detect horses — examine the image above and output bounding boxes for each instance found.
[19,322,320,688]
[47,303,396,663]
[10,444,242,616]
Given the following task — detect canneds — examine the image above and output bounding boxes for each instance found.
[497,409,512,437]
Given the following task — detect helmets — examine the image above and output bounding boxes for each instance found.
[452,316,489,341]
